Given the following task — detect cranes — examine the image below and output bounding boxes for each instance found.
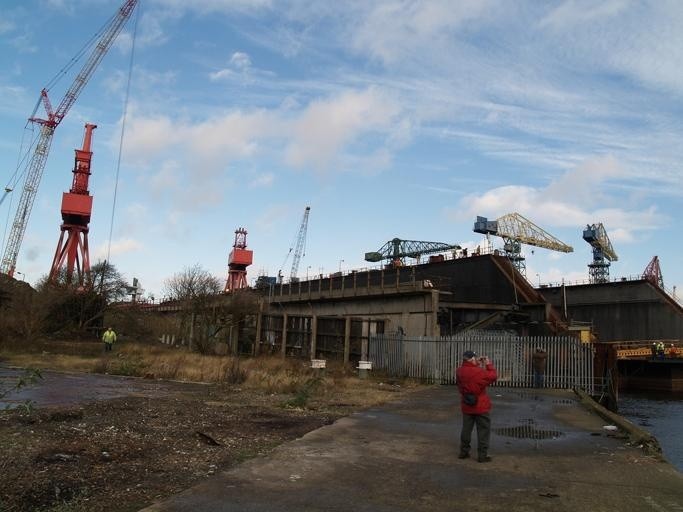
[353,236,460,271]
[279,205,314,282]
[581,222,618,287]
[469,207,577,284]
[0,1,141,277]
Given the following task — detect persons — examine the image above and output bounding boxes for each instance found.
[450,245,481,260]
[668,343,676,359]
[532,347,548,389]
[656,341,665,360]
[456,350,498,463]
[101,326,117,351]
[651,342,657,360]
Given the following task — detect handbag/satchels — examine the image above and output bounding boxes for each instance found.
[464,393,477,406]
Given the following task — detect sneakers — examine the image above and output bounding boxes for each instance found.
[478,455,492,462]
[458,451,472,458]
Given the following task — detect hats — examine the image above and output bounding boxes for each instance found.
[462,351,478,361]
